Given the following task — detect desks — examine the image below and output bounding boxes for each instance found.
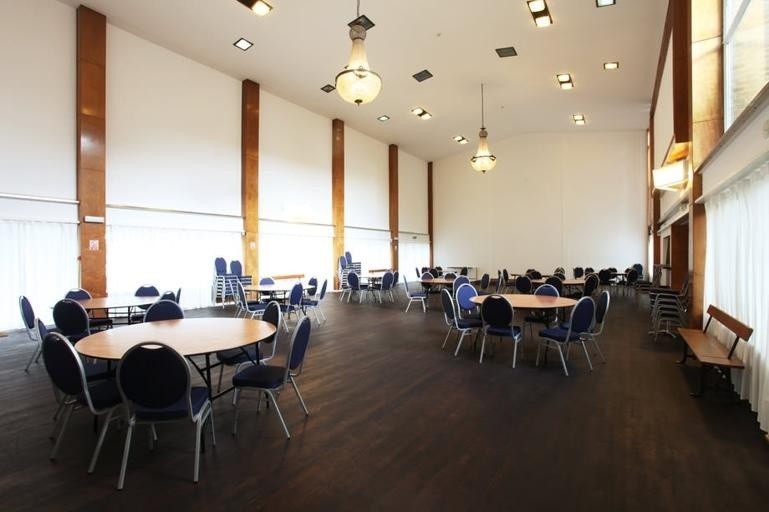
[418,276,470,292]
[469,294,579,339]
[531,276,590,295]
[243,283,316,311]
[76,317,277,410]
[70,295,161,330]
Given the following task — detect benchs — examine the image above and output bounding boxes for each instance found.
[676,305,754,398]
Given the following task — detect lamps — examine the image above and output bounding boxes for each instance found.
[331,2,385,110]
[467,83,497,176]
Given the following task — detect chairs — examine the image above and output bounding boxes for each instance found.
[231,315,311,439]
[35,318,111,421]
[337,252,691,378]
[16,256,327,372]
[115,340,218,491]
[215,300,282,394]
[40,332,128,473]
